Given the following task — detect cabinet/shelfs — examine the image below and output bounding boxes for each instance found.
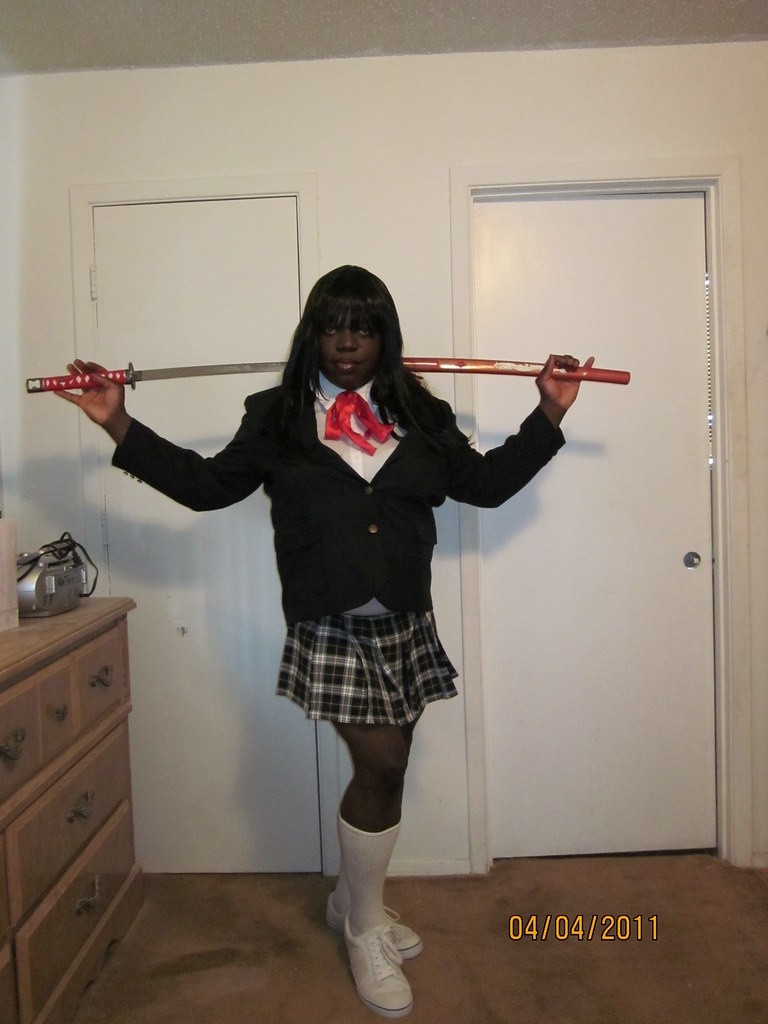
[0,595,146,1024]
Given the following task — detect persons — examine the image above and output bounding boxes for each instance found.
[54,264,595,1017]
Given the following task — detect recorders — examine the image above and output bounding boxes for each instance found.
[16,540,89,618]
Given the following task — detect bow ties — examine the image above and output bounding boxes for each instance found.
[324,392,396,457]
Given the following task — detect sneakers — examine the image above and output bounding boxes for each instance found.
[343,907,414,1018]
[325,890,423,959]
